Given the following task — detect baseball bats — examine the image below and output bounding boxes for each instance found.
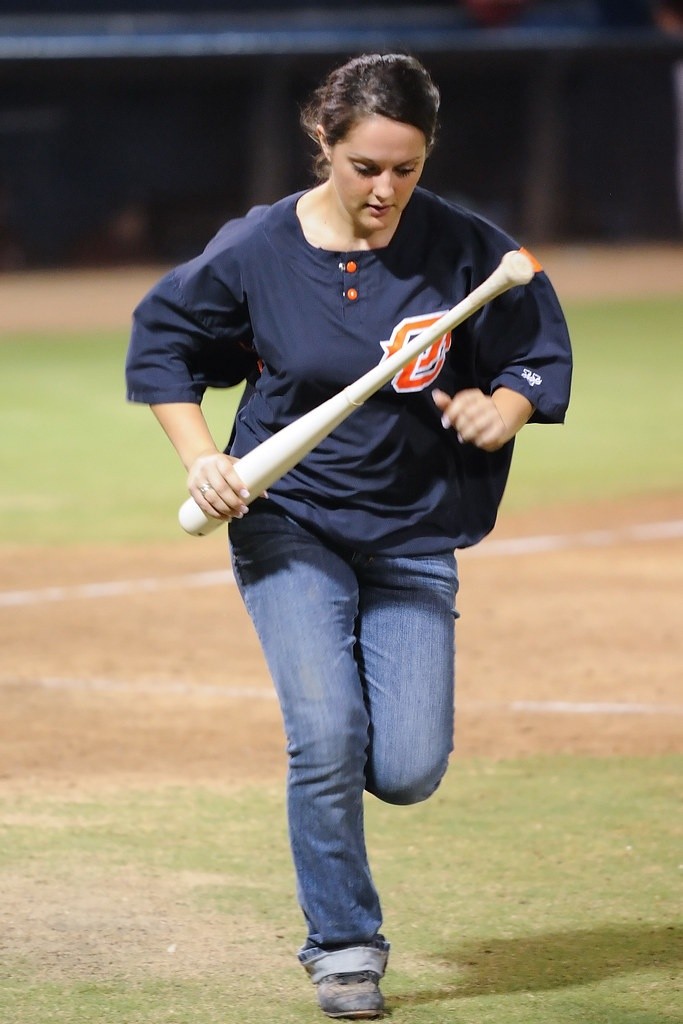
[178,250,535,537]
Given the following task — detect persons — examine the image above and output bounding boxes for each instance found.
[125,51,574,1019]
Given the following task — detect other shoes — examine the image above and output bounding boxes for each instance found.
[314,969,385,1019]
